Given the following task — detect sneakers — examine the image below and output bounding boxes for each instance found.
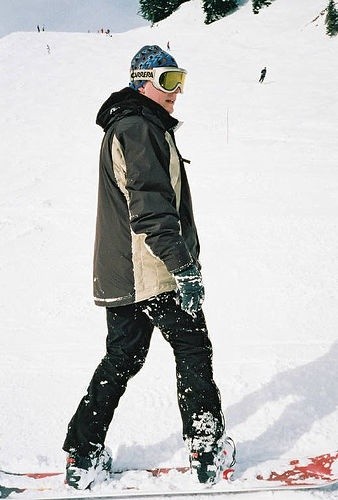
[66,447,112,490]
[189,437,236,487]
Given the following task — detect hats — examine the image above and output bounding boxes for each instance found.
[129,45,177,92]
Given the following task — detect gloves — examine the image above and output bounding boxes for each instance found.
[174,264,205,312]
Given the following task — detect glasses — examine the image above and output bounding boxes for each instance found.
[131,67,187,93]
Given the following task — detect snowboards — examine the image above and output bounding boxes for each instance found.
[0,450,338,498]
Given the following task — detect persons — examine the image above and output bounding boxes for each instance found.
[36,23,47,32]
[46,44,50,54]
[259,67,266,83]
[64,44,238,490]
[167,41,171,51]
[88,27,112,38]
